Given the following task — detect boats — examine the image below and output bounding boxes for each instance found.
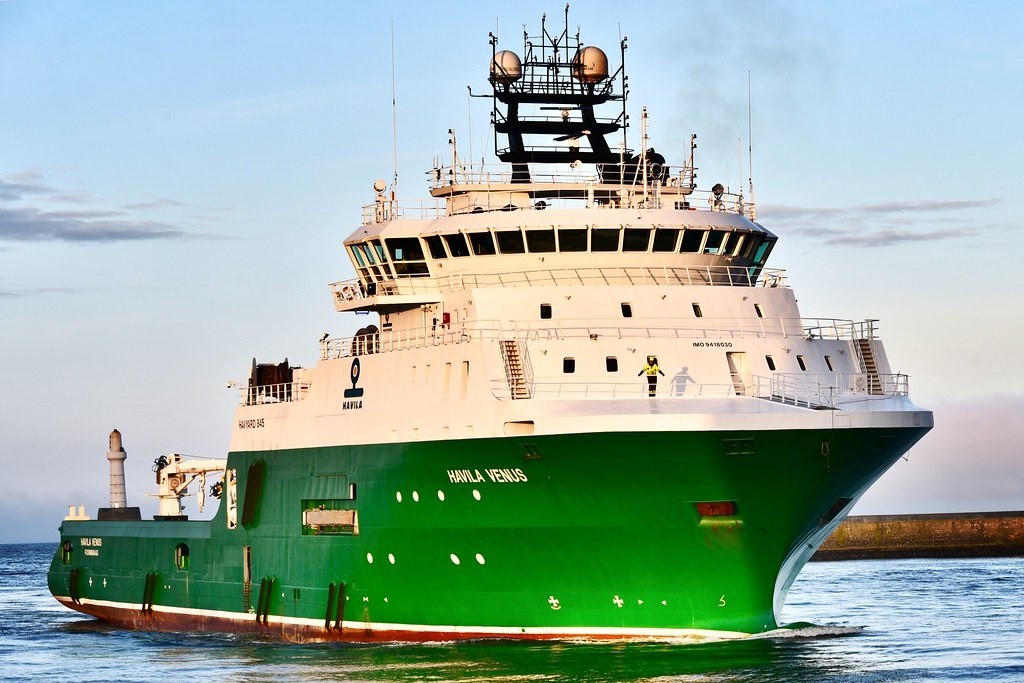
[47,3,934,644]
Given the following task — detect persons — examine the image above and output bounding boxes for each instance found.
[639,358,665,397]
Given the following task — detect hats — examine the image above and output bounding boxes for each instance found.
[649,357,654,361]
